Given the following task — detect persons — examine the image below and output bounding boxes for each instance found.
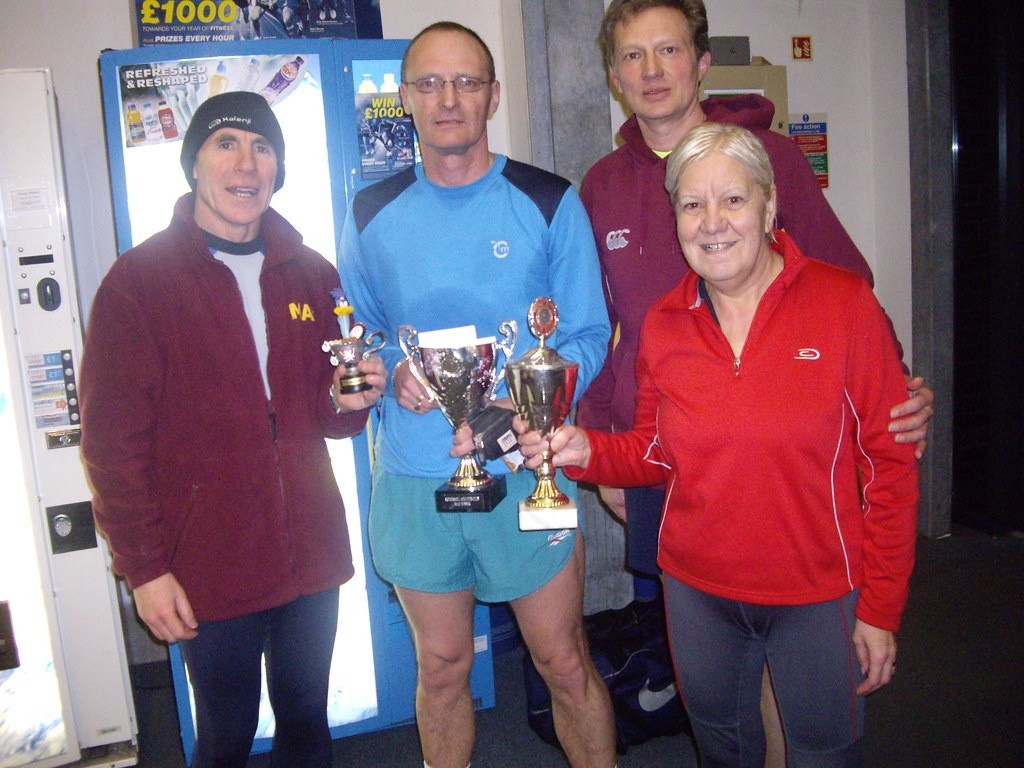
[511,123,920,768]
[361,118,412,159]
[338,21,616,768]
[232,0,353,41]
[80,91,386,768]
[574,0,933,768]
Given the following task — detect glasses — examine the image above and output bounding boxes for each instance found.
[405,75,495,93]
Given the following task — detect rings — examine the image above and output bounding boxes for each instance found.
[415,402,422,410]
[418,395,424,402]
[892,662,895,667]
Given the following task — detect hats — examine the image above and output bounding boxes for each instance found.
[181,91,286,193]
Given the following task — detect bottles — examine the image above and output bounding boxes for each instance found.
[381,74,398,92]
[187,85,197,116]
[258,56,304,105]
[170,98,187,130]
[158,101,178,138]
[234,60,260,90]
[143,103,162,140]
[127,104,146,143]
[197,83,208,106]
[359,73,377,93]
[208,61,229,97]
[177,91,192,125]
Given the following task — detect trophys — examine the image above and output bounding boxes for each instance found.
[504,296,579,532]
[398,319,517,513]
[322,288,388,395]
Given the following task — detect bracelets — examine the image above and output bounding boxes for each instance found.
[328,383,355,414]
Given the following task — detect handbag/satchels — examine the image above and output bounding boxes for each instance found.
[521,607,687,753]
